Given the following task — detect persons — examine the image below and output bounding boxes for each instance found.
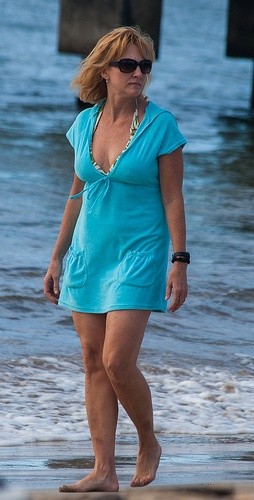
[44,27,188,492]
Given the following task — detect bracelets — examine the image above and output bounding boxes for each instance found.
[171,252,191,264]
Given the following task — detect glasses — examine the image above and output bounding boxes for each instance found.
[107,58,153,75]
